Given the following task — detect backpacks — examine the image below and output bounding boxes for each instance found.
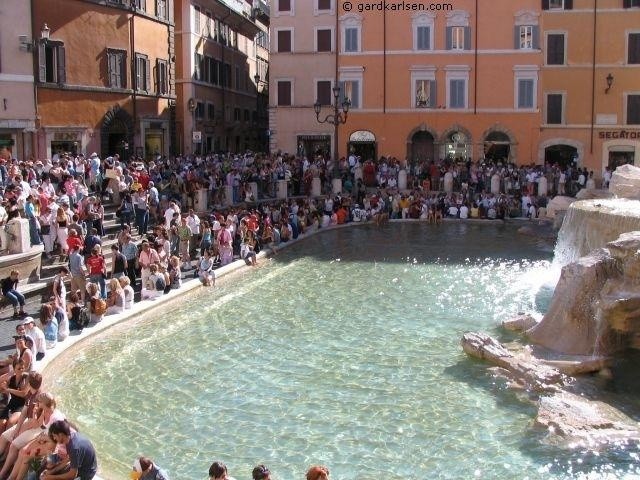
[77,307,90,326]
[95,298,107,315]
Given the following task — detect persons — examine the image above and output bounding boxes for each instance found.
[252,465,270,479]
[130,457,165,479]
[208,462,227,479]
[0,146,107,350]
[102,145,611,315]
[0,316,98,479]
[306,466,329,479]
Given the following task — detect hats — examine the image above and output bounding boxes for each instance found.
[22,317,33,325]
[130,457,150,480]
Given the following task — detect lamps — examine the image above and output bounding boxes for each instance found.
[37,23,51,47]
[254,72,269,85]
[605,73,613,93]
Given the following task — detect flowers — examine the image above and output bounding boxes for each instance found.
[25,447,45,480]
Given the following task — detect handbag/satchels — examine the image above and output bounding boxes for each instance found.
[157,278,164,290]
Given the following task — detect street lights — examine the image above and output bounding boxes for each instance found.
[312,86,355,179]
[254,72,261,92]
